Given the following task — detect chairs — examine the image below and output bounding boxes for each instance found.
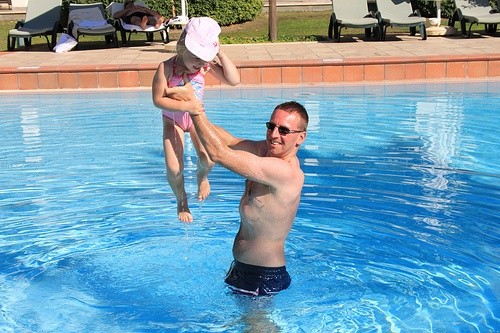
[7,0,67,52]
[328,0,382,43]
[105,0,171,47]
[64,3,119,49]
[448,0,500,39]
[373,0,427,41]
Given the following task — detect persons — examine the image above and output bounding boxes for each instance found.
[113,0,164,29]
[152,18,240,222]
[164,72,309,296]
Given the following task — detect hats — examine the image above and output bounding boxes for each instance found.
[184,15,222,61]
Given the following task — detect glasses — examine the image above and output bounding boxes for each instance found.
[265,121,303,135]
